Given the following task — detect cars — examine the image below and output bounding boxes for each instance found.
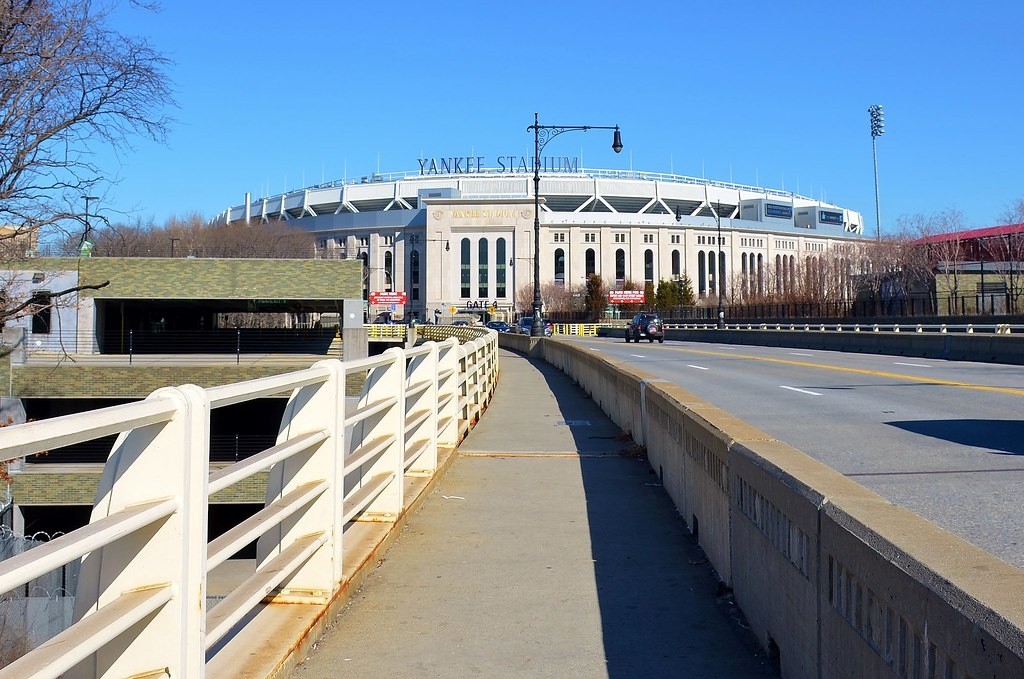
[450,320,469,327]
[516,316,553,337]
[371,312,435,325]
[486,321,511,334]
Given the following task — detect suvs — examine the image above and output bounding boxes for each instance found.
[624,313,665,344]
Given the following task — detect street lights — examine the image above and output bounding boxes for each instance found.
[866,103,885,242]
[169,238,181,257]
[532,112,624,336]
[409,231,451,330]
[80,195,100,243]
[674,198,726,328]
